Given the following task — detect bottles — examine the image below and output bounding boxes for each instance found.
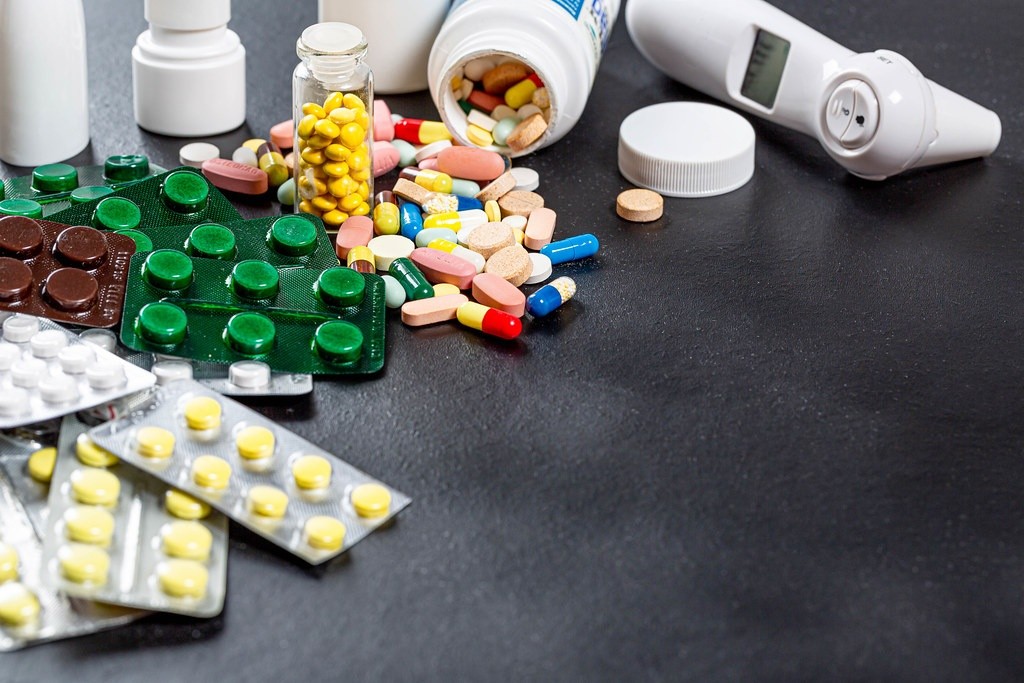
[319,0,449,94]
[0,0,89,166]
[292,22,376,232]
[427,0,622,159]
